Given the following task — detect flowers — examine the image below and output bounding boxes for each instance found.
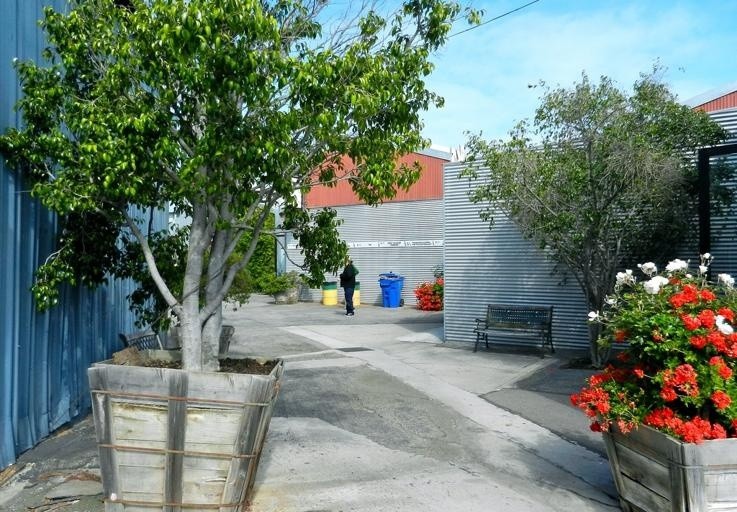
[571,253,737,445]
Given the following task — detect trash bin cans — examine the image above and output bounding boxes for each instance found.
[379,273,404,308]
[321,282,360,307]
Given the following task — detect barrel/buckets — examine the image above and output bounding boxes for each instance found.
[321,281,337,306]
[352,281,361,307]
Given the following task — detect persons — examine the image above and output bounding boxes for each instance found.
[340,260,355,315]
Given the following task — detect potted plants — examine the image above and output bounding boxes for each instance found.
[256,270,303,305]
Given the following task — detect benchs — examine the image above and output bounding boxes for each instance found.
[119,331,182,351]
[473,304,555,359]
